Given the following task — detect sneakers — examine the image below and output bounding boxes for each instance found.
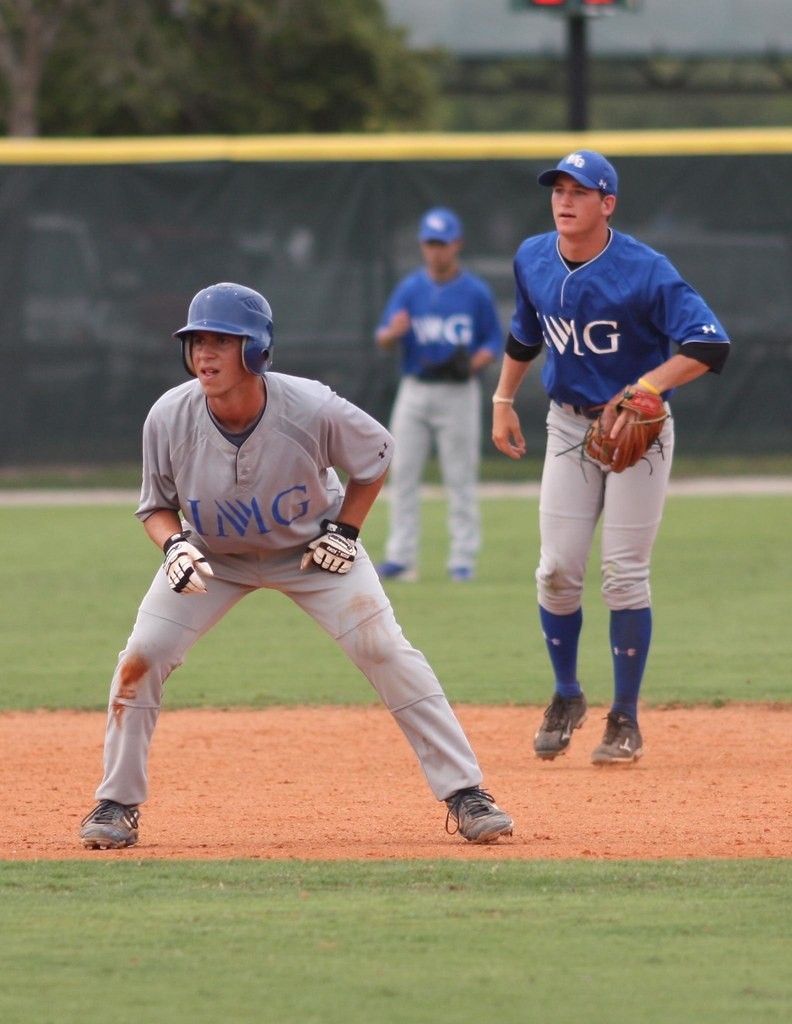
[591,713,643,763]
[532,679,587,758]
[79,799,142,849]
[443,785,515,842]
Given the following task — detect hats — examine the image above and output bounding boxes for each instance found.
[416,208,462,242]
[537,149,619,196]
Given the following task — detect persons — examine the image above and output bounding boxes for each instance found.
[371,207,501,580]
[492,150,731,764]
[80,283,513,850]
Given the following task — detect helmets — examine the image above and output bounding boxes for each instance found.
[172,282,274,375]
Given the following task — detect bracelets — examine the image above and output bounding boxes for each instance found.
[493,395,514,404]
[637,379,661,395]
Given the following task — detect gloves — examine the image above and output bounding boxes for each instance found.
[300,518,360,573]
[162,530,214,595]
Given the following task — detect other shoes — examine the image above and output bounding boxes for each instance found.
[375,562,419,582]
[448,566,474,580]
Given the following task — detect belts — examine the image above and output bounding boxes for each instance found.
[561,402,603,419]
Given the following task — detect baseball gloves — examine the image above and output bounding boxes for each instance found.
[580,384,670,474]
